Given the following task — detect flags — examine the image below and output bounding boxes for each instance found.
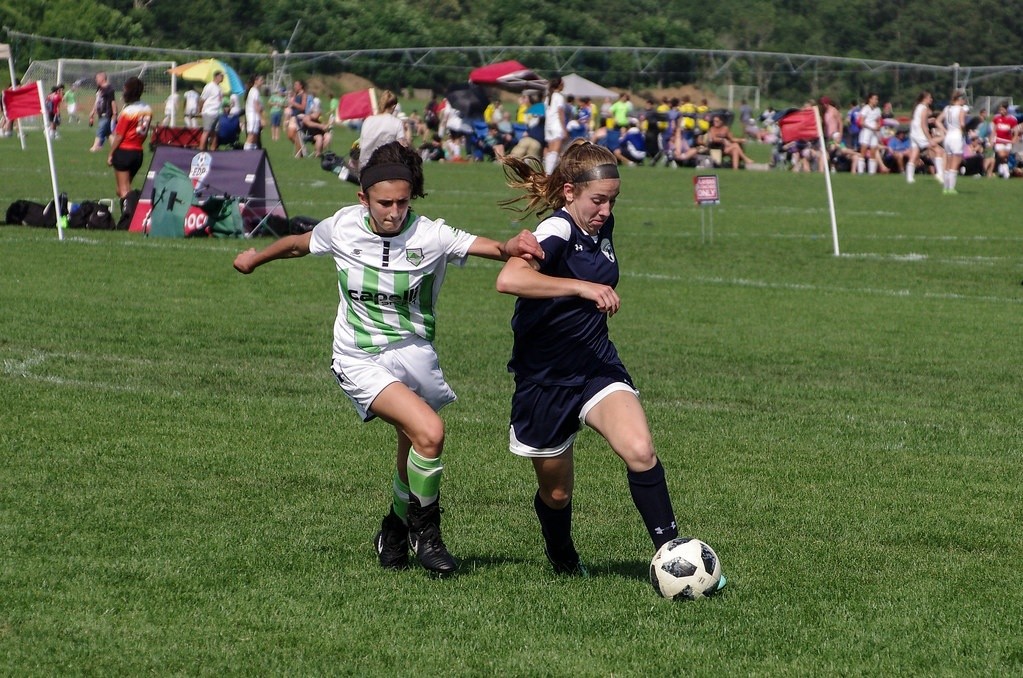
[2,82,43,122]
[778,108,818,143]
[339,89,373,118]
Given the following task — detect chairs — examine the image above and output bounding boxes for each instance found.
[294,126,318,160]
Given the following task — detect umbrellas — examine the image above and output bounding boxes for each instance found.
[449,86,487,112]
[471,59,618,98]
[168,57,246,94]
[523,102,545,117]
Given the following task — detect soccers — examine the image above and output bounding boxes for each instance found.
[649,538,721,603]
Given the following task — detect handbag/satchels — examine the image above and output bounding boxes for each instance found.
[3,190,140,231]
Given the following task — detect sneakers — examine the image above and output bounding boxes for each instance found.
[714,576,726,590]
[374,508,410,572]
[407,518,458,573]
[544,543,586,577]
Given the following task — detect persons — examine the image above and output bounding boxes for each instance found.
[327,93,362,130]
[233,141,544,574]
[242,71,333,158]
[198,71,224,151]
[357,89,545,171]
[108,77,153,198]
[216,94,245,148]
[183,85,200,128]
[563,88,1023,195]
[0,78,22,137]
[89,72,116,152]
[495,138,726,591]
[162,90,180,128]
[46,83,77,140]
